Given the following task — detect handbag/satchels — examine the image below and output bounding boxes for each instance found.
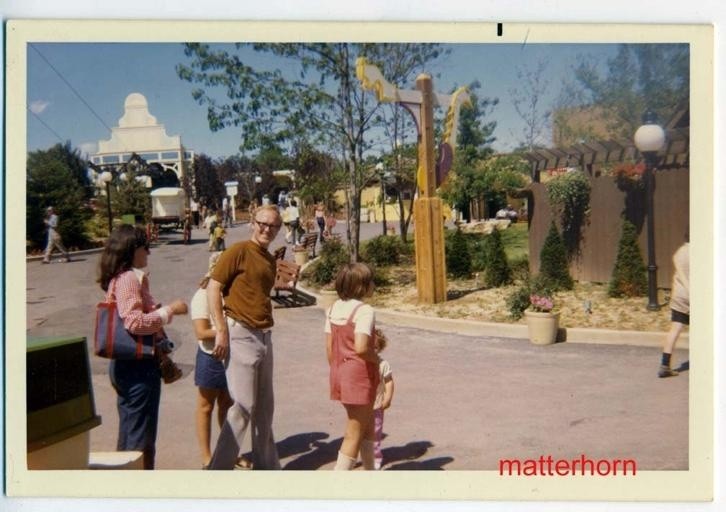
[94,302,156,360]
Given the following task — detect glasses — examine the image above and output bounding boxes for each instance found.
[255,220,280,231]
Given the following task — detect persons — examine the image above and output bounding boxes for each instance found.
[281,199,328,243]
[371,329,394,471]
[38,206,70,264]
[214,224,225,249]
[186,250,251,471]
[201,209,217,251]
[654,228,689,379]
[206,203,284,471]
[94,223,189,469]
[322,261,382,471]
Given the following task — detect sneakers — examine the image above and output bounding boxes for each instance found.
[658,366,679,377]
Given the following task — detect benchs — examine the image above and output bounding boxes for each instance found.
[273,233,318,303]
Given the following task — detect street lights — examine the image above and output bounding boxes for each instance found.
[99,164,115,235]
[119,172,131,187]
[375,158,390,234]
[252,174,262,208]
[633,109,669,314]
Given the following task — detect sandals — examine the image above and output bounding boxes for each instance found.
[235,457,255,469]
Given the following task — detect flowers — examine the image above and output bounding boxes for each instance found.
[529,294,554,313]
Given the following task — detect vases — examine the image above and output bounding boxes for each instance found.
[523,309,561,346]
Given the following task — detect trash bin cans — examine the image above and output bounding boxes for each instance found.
[120,213,144,227]
[26,331,102,470]
